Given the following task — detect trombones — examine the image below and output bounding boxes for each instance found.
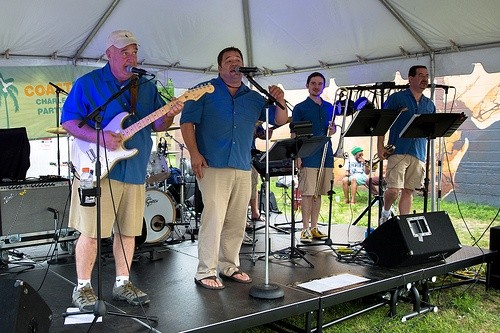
[313,86,350,203]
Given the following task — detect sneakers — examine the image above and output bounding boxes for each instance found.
[71,283,98,313]
[112,280,150,305]
[309,227,328,238]
[300,229,313,242]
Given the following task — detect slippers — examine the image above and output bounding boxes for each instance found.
[194,277,225,289]
[219,268,252,283]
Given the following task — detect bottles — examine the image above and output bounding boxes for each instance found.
[80,168,96,203]
[365,227,375,238]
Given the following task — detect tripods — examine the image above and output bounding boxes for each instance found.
[167,136,196,239]
[61,73,160,333]
[250,136,350,268]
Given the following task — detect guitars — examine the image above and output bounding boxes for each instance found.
[68,82,217,182]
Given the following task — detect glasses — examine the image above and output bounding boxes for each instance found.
[359,154,363,156]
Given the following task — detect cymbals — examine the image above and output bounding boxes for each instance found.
[151,126,181,132]
[44,127,69,134]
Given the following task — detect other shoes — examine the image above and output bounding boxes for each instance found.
[380,212,391,225]
[242,232,253,245]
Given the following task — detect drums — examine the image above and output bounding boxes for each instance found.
[135,151,176,243]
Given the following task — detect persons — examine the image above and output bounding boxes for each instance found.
[180,47,289,290]
[292,71,337,243]
[343,65,436,223]
[60,29,185,315]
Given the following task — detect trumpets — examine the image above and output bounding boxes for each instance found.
[364,143,396,175]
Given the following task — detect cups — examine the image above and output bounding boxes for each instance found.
[335,196,340,202]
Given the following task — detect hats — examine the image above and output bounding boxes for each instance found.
[351,147,363,156]
[106,31,141,49]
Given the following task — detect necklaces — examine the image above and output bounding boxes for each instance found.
[225,83,240,89]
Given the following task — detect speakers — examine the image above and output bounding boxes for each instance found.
[0,179,71,236]
[0,279,53,333]
[361,211,462,268]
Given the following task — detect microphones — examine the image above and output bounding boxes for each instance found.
[126,65,154,76]
[235,66,258,73]
[157,138,162,151]
[154,221,163,227]
[47,207,59,214]
[431,83,454,88]
